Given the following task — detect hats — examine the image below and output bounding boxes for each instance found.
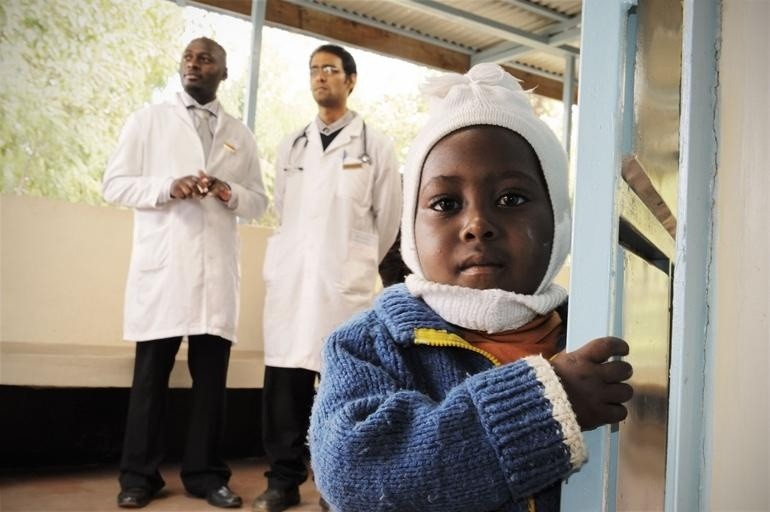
[401,63,570,332]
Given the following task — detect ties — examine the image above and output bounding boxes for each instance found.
[194,108,213,158]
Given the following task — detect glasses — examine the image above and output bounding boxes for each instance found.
[310,66,339,76]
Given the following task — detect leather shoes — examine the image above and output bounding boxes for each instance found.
[208,485,242,508]
[255,485,301,512]
[117,487,149,508]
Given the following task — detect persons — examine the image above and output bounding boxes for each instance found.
[96,35,271,510]
[246,42,408,511]
[306,58,637,509]
